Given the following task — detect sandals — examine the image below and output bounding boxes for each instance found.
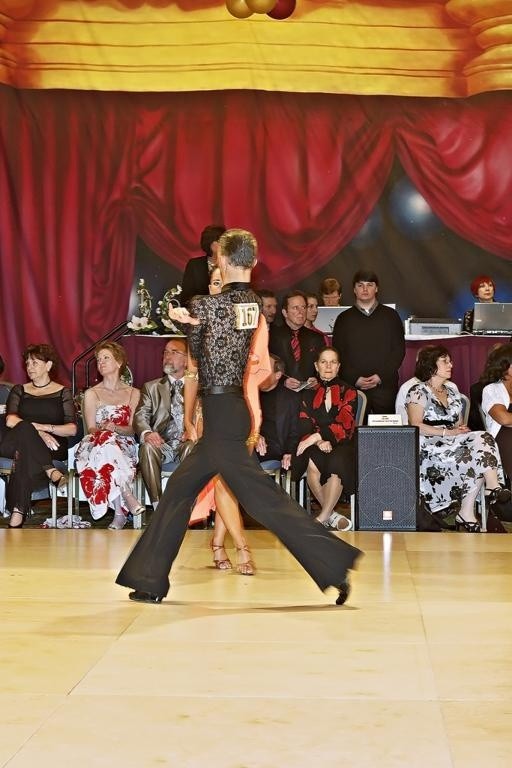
[107,511,128,531]
[123,492,147,516]
[209,536,233,570]
[327,508,354,532]
[234,543,256,577]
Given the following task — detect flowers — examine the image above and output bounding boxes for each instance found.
[124,275,187,336]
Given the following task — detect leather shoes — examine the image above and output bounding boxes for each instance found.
[127,588,165,604]
[332,548,366,607]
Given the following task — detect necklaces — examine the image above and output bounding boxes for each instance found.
[32,379,51,388]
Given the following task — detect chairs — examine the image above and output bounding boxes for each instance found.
[2,380,511,535]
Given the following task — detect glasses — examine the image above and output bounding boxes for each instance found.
[163,349,187,357]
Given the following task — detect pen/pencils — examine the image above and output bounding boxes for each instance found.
[283,374,302,386]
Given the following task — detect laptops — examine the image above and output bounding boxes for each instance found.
[473,302,511,334]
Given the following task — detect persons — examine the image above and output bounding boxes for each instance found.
[170,223,408,533]
[76,339,147,532]
[181,267,272,574]
[115,229,366,606]
[462,273,508,335]
[132,337,188,513]
[395,336,512,533]
[1,344,80,529]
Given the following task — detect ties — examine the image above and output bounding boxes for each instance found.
[170,380,186,452]
[289,329,301,363]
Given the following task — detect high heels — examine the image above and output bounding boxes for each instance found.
[49,469,67,494]
[485,487,512,508]
[454,512,489,533]
[7,510,27,529]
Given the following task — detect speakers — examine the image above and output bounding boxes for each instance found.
[354,425,418,533]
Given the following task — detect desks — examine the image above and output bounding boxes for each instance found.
[112,331,512,400]
